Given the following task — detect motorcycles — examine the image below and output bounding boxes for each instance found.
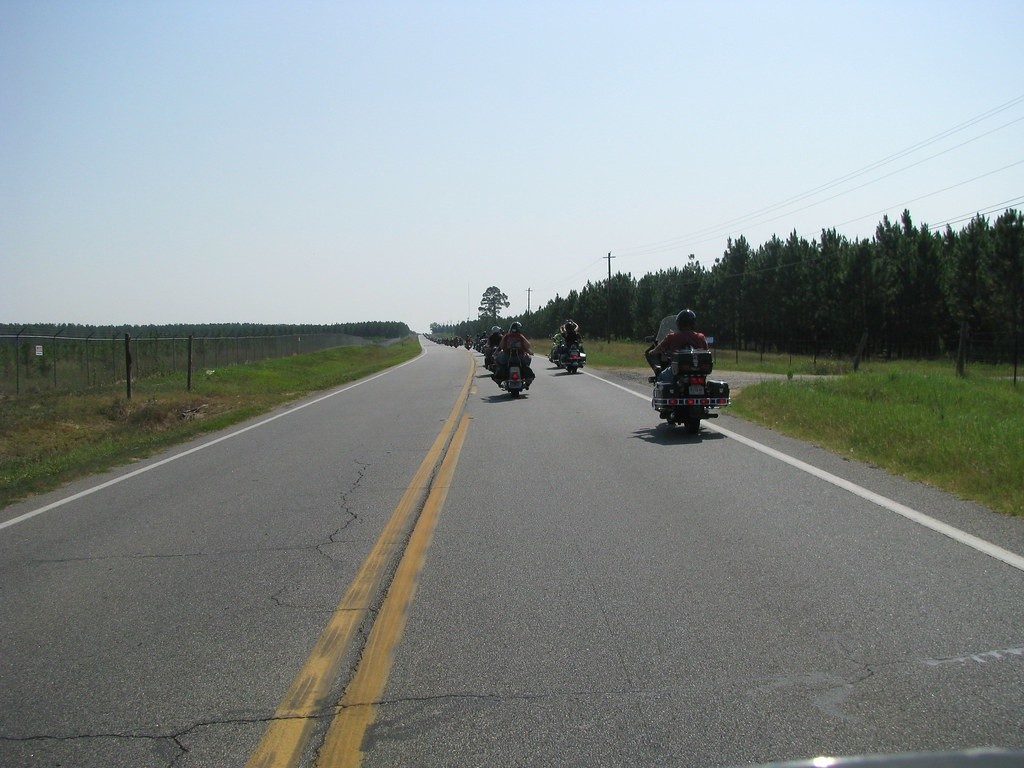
[438,337,535,398]
[545,332,587,374]
[643,314,731,435]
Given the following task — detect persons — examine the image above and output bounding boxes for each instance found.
[424,322,535,390]
[649,309,709,419]
[550,319,582,366]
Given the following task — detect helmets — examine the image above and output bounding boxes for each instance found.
[509,321,523,333]
[565,322,575,331]
[492,326,500,334]
[676,309,696,331]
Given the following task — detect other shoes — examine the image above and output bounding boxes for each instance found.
[490,374,501,385]
[660,403,675,419]
[523,383,529,390]
